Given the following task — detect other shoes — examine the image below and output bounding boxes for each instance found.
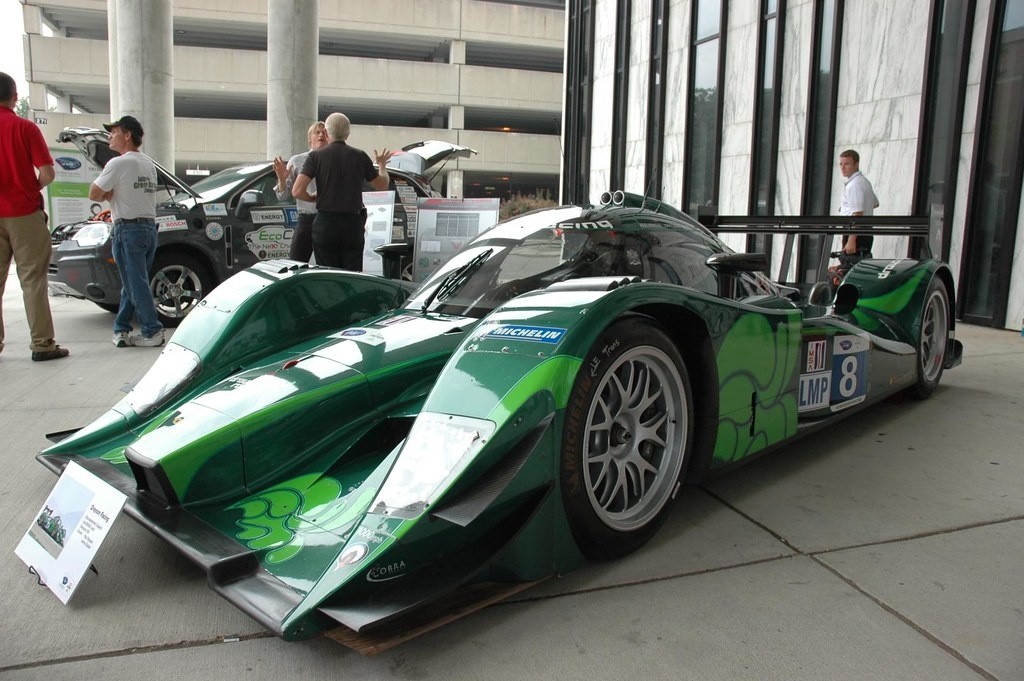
[32,345,69,361]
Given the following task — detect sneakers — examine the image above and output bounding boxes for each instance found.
[113,331,130,347]
[129,331,165,346]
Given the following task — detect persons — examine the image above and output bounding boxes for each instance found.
[292,112,393,272]
[0,72,69,361]
[272,121,328,263]
[838,150,879,266]
[88,114,167,347]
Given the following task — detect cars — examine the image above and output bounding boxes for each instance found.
[58,126,479,329]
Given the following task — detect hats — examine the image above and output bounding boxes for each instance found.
[103,116,143,137]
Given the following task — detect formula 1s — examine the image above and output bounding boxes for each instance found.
[32,191,964,643]
